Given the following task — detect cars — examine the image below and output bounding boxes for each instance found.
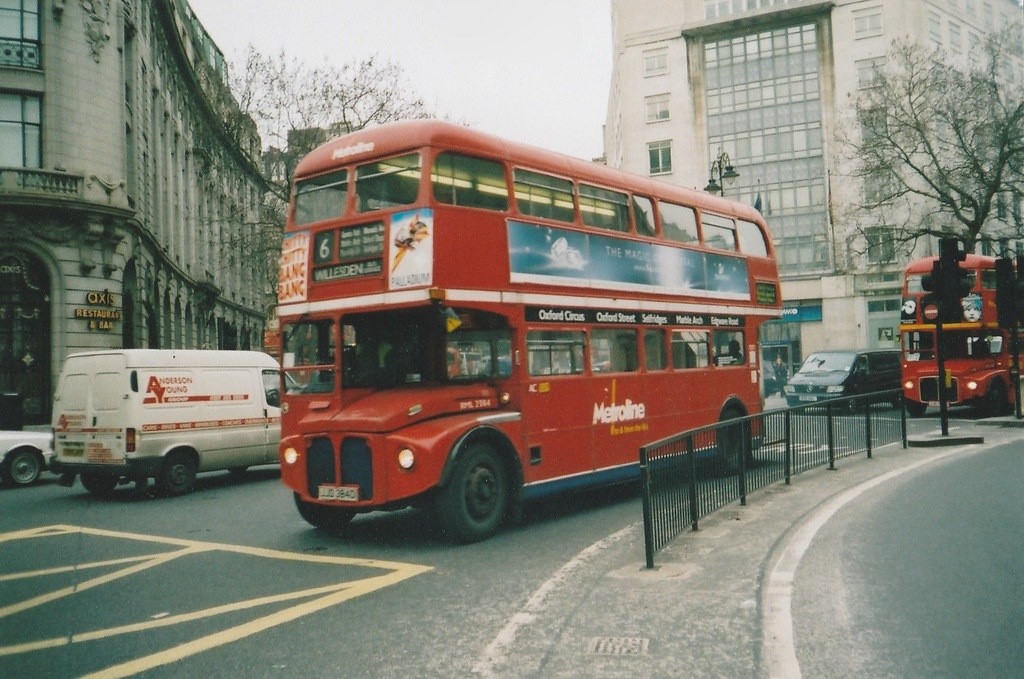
[0,428,55,487]
[780,346,905,416]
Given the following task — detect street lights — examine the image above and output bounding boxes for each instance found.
[703,152,740,197]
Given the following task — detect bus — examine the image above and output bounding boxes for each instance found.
[898,250,1024,422]
[261,117,783,549]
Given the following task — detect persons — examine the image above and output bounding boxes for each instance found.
[775,359,788,398]
[727,340,744,366]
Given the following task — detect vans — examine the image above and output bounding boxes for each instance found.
[46,344,307,499]
